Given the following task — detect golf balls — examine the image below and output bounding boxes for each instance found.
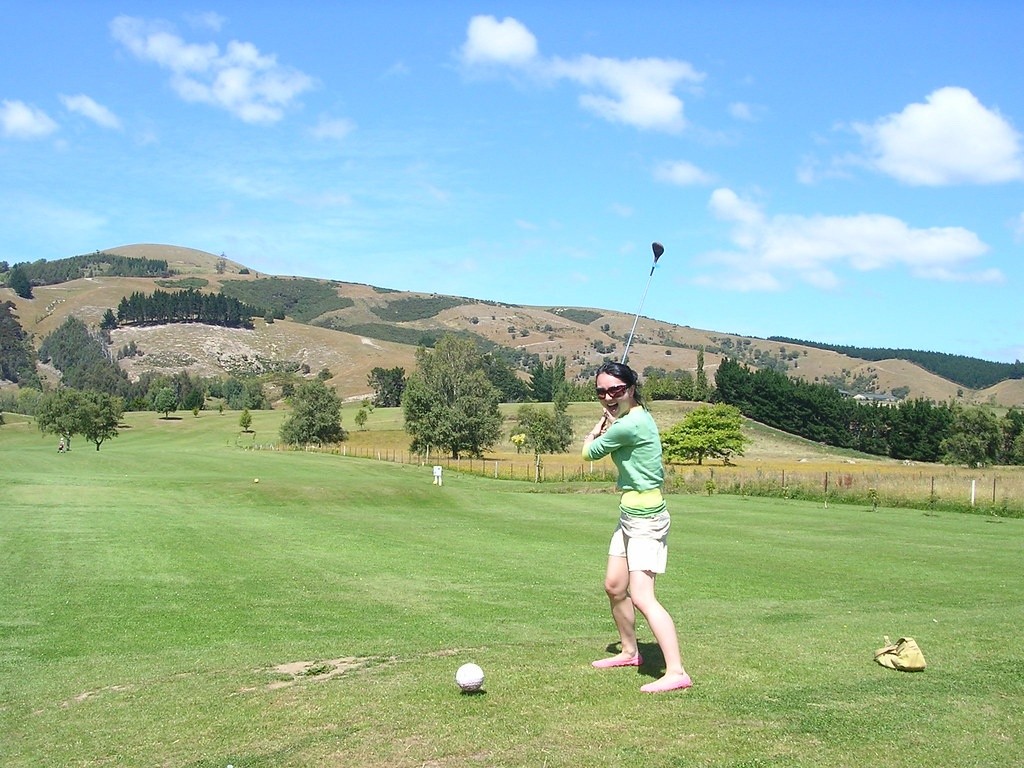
[455,663,484,694]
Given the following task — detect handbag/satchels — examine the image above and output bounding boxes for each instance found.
[873,636,928,672]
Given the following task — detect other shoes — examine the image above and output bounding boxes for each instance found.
[641,674,693,693]
[591,652,644,668]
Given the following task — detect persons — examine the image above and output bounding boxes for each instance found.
[582,361,693,693]
[57,438,64,453]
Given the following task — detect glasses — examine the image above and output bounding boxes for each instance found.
[595,384,629,400]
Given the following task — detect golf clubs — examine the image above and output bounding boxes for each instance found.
[600,240,664,428]
